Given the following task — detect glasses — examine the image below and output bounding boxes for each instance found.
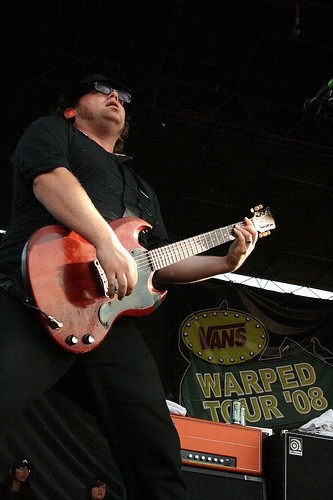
[92,83,132,103]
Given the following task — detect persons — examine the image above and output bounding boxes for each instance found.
[7,455,33,500]
[1,76,260,500]
[85,468,108,500]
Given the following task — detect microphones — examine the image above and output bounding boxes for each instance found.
[304,79,333,115]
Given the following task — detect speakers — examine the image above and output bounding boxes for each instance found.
[261,430,333,500]
[178,466,267,500]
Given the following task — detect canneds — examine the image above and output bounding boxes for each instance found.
[240,407,247,426]
[229,400,241,424]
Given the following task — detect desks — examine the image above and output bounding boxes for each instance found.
[181,466,267,500]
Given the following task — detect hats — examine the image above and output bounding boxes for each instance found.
[77,74,133,107]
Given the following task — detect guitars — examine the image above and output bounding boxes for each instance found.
[22,205,276,355]
